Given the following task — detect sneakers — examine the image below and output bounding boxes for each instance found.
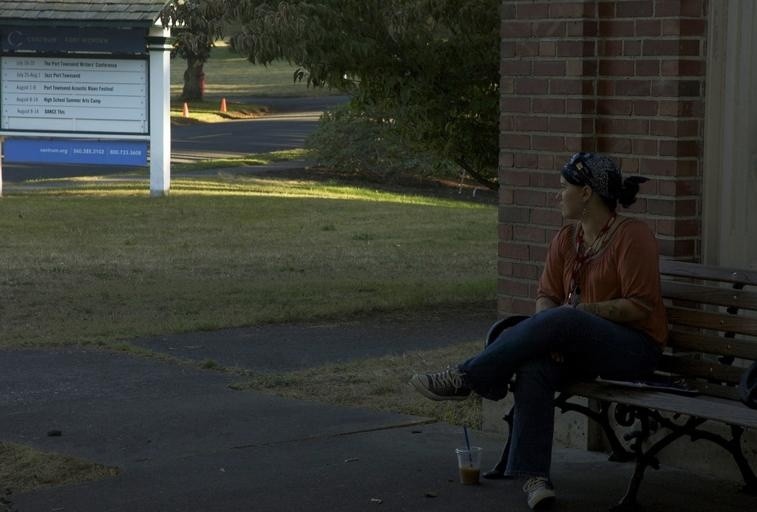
[522,476,555,508]
[410,365,471,401]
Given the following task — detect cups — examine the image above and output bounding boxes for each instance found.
[455,447,483,484]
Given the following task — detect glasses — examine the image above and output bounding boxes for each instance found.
[569,151,600,188]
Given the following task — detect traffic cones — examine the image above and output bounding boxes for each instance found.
[219,97,227,113]
[182,102,189,118]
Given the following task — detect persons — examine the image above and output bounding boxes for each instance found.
[409,149,668,511]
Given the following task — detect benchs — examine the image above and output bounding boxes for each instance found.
[483,258,756,508]
